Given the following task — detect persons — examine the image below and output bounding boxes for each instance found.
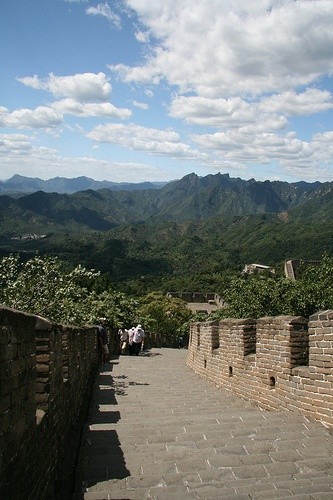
[97,318,110,364]
[118,325,136,355]
[129,325,145,356]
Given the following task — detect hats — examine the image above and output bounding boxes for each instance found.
[132,327,135,329]
[137,325,141,329]
[99,318,106,322]
